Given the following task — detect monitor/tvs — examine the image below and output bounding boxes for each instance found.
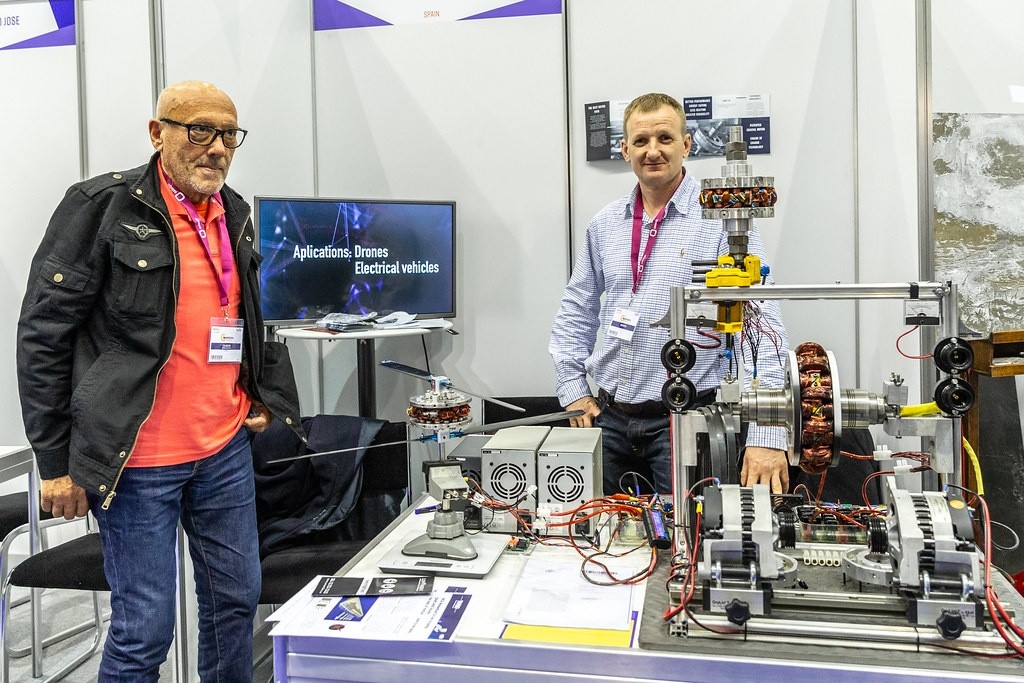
[253,196,456,325]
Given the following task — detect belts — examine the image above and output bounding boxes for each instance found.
[597,387,716,420]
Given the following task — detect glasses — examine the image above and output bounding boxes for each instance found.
[160,117,248,148]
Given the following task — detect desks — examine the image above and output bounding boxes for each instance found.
[274,318,454,419]
[272,488,1024,683]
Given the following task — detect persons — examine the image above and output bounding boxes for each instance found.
[15,80,271,683]
[548,92,793,499]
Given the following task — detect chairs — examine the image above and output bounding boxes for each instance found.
[177,416,414,683]
[480,397,884,507]
[0,455,112,683]
